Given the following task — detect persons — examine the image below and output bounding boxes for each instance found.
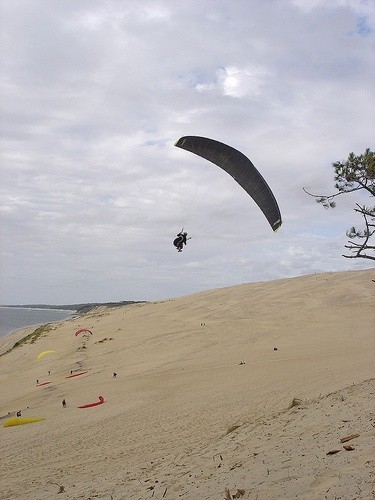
[35,323,102,390]
[113,372,118,378]
[16,410,22,418]
[62,398,67,407]
[172,231,189,252]
